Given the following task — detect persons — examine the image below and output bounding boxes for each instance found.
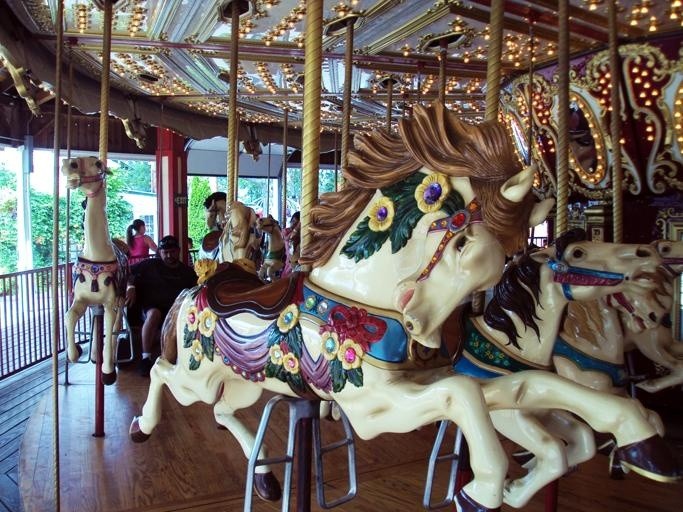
[258,216,264,237]
[125,235,198,376]
[281,212,299,276]
[125,219,158,265]
[187,238,194,270]
[203,192,229,229]
